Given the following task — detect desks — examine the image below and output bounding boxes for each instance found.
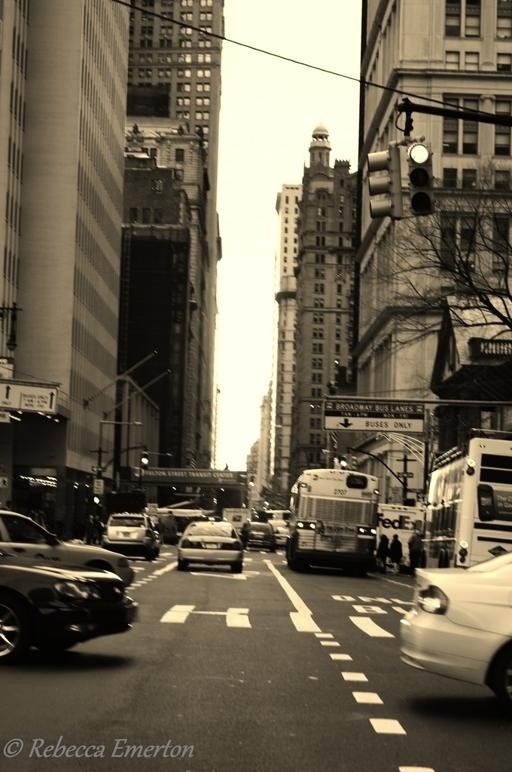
[94,420,142,514]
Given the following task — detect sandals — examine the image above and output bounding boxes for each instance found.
[422,436,511,569]
[289,468,380,578]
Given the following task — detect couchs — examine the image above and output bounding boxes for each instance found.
[339,457,347,469]
[141,452,148,470]
[408,143,436,216]
[366,150,402,220]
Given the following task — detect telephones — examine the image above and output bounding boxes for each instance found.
[322,399,425,434]
[0,382,59,416]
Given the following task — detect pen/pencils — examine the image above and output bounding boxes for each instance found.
[376,502,424,551]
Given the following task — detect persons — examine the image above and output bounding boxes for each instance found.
[407,533,425,572]
[389,535,404,574]
[375,535,388,570]
[85,515,104,545]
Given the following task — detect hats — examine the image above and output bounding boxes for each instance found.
[399,552,511,703]
[0,552,139,663]
[102,512,160,560]
[0,510,134,588]
[177,521,244,573]
[246,521,288,551]
